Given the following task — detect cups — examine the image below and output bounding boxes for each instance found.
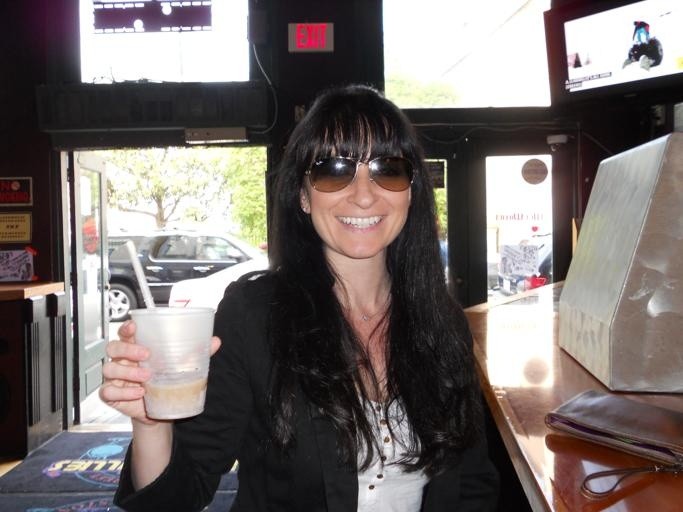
[126,307,215,420]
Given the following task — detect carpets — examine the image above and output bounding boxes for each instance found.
[0,431,240,512]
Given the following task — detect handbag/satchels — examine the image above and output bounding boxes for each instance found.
[544,387,682,500]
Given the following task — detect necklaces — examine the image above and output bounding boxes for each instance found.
[357,297,390,322]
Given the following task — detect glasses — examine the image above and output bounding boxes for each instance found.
[304,155,421,194]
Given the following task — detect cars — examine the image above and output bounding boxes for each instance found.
[97,234,268,322]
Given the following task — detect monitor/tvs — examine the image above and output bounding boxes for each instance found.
[560,0,683,93]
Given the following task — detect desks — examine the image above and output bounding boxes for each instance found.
[0,280,73,458]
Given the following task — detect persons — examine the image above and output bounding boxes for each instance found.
[631,19,651,46]
[96,85,503,509]
